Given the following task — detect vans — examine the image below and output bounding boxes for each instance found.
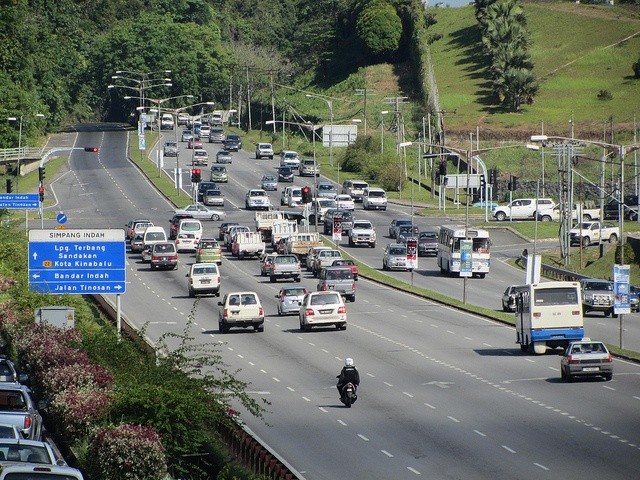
[178,218,203,241]
[228,134,242,149]
[177,112,189,127]
[192,149,208,167]
[364,189,387,210]
[279,150,300,169]
[144,227,167,248]
[159,113,174,130]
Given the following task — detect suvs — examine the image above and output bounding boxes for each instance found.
[256,143,274,160]
[246,188,271,209]
[397,224,419,243]
[305,247,331,271]
[288,187,304,207]
[492,197,555,221]
[598,195,638,220]
[298,158,320,177]
[324,208,353,238]
[580,279,618,319]
[198,125,211,139]
[316,266,355,303]
[209,114,223,126]
[348,219,376,248]
[208,128,225,143]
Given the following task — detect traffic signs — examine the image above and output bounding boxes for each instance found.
[6,179,13,193]
[28,229,127,295]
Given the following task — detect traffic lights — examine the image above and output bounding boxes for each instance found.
[301,186,312,203]
[192,169,196,182]
[85,147,98,152]
[39,187,45,202]
[510,175,519,191]
[196,169,201,182]
[39,167,46,181]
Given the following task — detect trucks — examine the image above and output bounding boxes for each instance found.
[214,109,241,127]
[230,226,264,260]
[287,232,318,254]
[256,208,285,235]
[270,219,299,243]
[215,291,264,333]
[304,197,337,226]
[341,179,368,202]
[185,263,221,296]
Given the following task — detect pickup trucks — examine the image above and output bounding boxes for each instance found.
[333,259,358,280]
[569,222,620,245]
[417,231,439,256]
[312,249,342,274]
[534,201,600,222]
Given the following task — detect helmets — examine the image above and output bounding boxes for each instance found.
[345,358,353,365]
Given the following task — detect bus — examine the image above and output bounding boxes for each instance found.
[514,281,585,355]
[437,224,491,280]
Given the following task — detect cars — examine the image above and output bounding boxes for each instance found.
[0,438,57,467]
[198,183,217,202]
[151,243,179,272]
[612,285,640,311]
[389,219,414,238]
[209,164,228,182]
[382,244,410,273]
[274,286,308,315]
[561,340,613,381]
[193,124,201,133]
[203,188,224,205]
[142,248,151,262]
[224,140,238,151]
[175,233,199,252]
[269,255,303,282]
[182,130,192,142]
[0,423,22,440]
[175,204,227,221]
[218,222,238,238]
[194,241,222,265]
[320,184,336,200]
[188,138,202,149]
[0,381,40,434]
[260,175,277,191]
[0,466,83,480]
[502,285,519,312]
[281,187,290,206]
[132,222,152,233]
[164,140,177,158]
[0,359,17,382]
[336,194,355,212]
[215,151,233,165]
[130,235,145,251]
[260,255,273,276]
[223,226,234,243]
[297,291,348,332]
[279,170,293,182]
[126,218,151,239]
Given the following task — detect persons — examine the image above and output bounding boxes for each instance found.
[337,357,360,406]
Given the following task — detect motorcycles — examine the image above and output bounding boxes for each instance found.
[336,374,357,409]
[277,245,285,254]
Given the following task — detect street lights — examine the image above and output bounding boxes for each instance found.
[381,111,407,180]
[531,135,640,349]
[150,109,237,204]
[124,95,193,178]
[0,118,16,120]
[136,102,215,196]
[398,141,539,304]
[265,119,362,232]
[112,76,172,134]
[17,114,44,177]
[116,70,171,109]
[108,83,172,160]
[306,94,333,167]
